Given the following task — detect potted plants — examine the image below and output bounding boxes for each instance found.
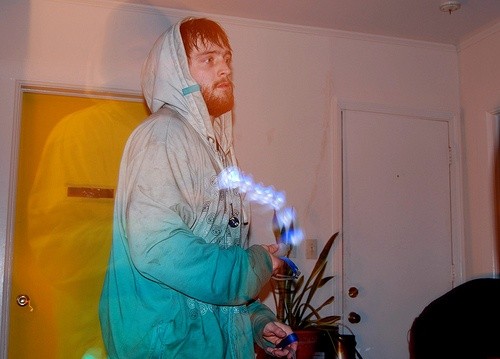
[254,206,340,359]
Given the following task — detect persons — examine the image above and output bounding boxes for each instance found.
[406,276,500,358]
[99,16,301,359]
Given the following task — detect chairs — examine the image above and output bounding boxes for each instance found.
[407,277,500,359]
